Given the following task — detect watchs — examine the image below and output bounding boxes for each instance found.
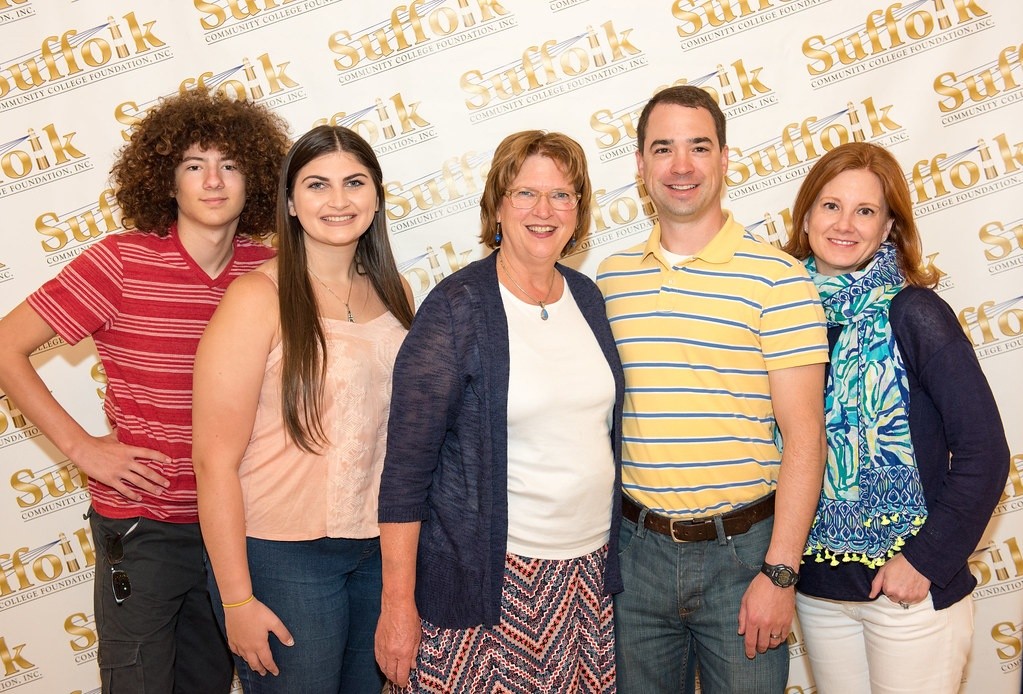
[762,561,798,588]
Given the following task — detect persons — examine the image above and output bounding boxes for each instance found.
[596,86,830,694]
[375,130,625,694]
[0,91,409,694]
[774,143,1011,694]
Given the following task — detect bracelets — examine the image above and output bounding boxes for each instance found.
[222,594,254,608]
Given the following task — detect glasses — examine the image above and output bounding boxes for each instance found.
[105,534,132,604]
[504,188,581,210]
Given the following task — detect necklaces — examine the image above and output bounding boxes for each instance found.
[500,252,555,320]
[306,264,355,323]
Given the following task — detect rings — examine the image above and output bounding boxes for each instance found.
[770,633,782,638]
[899,600,910,609]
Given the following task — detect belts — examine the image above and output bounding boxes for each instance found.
[622,492,775,543]
